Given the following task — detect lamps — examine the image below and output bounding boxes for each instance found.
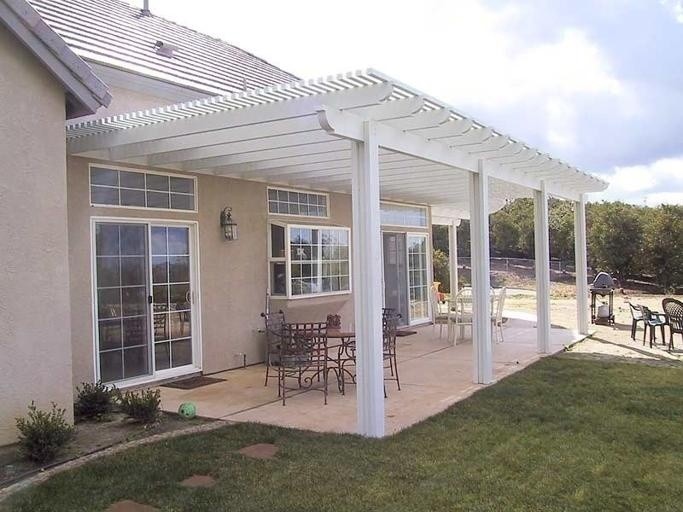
[219,206,236,240]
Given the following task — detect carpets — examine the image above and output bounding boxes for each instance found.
[158,375,227,390]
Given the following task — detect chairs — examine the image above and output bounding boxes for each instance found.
[428,285,507,347]
[624,298,683,352]
[120,304,167,351]
[260,307,402,406]
